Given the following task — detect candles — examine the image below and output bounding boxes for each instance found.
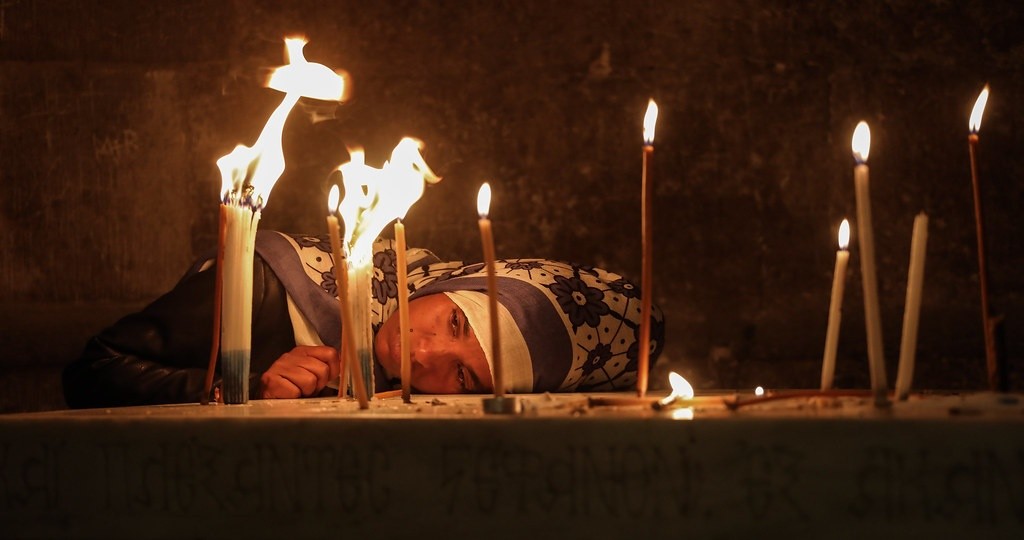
[202,36,444,408]
[726,390,918,409]
[474,181,503,396]
[968,84,1005,390]
[652,371,695,411]
[849,120,894,406]
[822,219,850,390]
[895,209,929,397]
[636,98,658,392]
[583,392,738,409]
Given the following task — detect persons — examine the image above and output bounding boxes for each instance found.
[63,230,666,409]
